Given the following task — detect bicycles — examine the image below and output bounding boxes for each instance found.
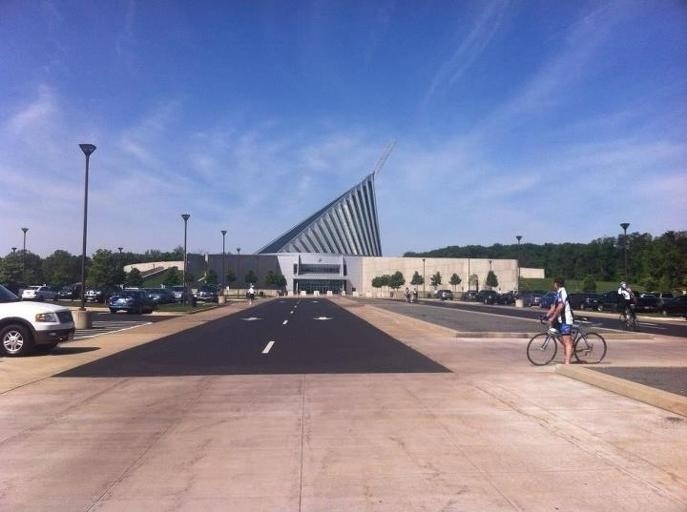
[619,304,638,331]
[247,295,258,306]
[527,312,606,366]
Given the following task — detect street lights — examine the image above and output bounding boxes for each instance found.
[181,213,191,305]
[515,235,524,307]
[117,247,123,253]
[217,230,226,304]
[236,247,241,297]
[12,247,17,252]
[21,227,29,287]
[620,223,631,287]
[422,257,426,297]
[488,260,494,292]
[76,144,97,328]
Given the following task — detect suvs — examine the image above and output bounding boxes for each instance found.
[461,290,479,302]
[434,290,453,301]
[0,282,75,357]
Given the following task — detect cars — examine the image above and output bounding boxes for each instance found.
[107,290,156,314]
[476,289,687,319]
[194,286,219,303]
[18,283,195,303]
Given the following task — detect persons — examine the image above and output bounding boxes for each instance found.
[537,277,575,365]
[404,287,411,302]
[412,287,418,302]
[616,281,640,329]
[248,284,258,306]
